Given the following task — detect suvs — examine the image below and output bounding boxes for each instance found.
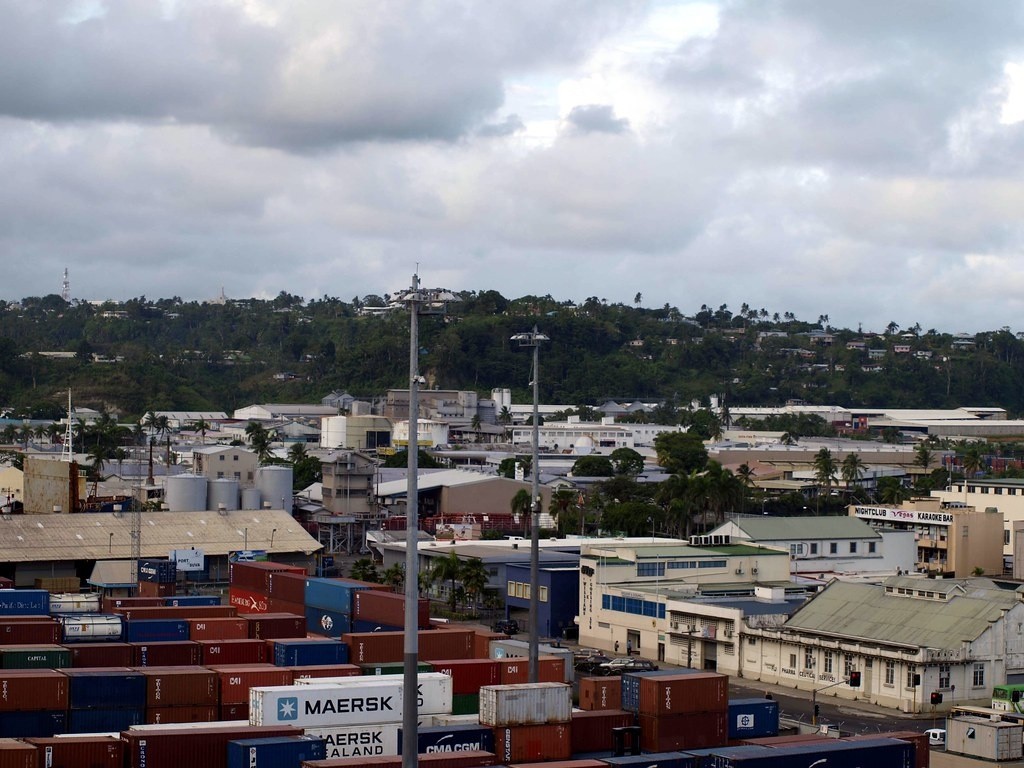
[490,620,520,635]
[574,648,604,659]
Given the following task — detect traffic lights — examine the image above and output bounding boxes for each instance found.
[851,672,862,688]
[929,691,943,704]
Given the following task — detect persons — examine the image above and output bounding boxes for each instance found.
[627,640,632,656]
[549,637,561,647]
[765,691,772,700]
[614,641,619,655]
[598,651,603,656]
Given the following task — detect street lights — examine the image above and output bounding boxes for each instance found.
[511,325,552,683]
[384,272,466,768]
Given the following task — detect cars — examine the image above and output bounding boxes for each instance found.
[599,656,636,676]
[575,656,614,673]
[612,658,661,676]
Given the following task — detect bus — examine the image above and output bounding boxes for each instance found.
[992,683,1024,713]
